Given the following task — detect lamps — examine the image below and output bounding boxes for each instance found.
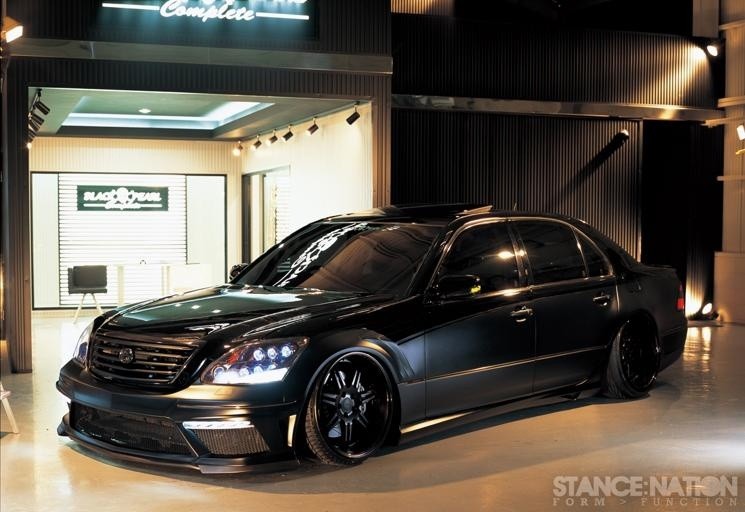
[231,100,359,157]
[736,123,745,141]
[0,16,24,44]
[701,302,719,320]
[707,41,724,56]
[27,88,50,150]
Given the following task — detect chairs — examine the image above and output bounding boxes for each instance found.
[437,232,495,292]
[67,265,108,325]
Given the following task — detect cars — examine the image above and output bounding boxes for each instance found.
[56,205,687,475]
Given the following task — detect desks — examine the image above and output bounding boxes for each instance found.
[114,263,171,307]
